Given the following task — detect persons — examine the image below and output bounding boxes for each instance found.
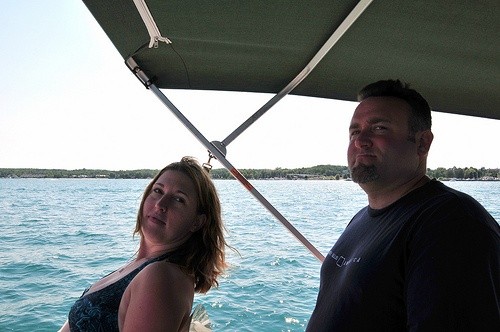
[55,156,244,331]
[304,77,500,332]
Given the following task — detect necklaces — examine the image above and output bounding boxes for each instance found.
[118,256,157,274]
